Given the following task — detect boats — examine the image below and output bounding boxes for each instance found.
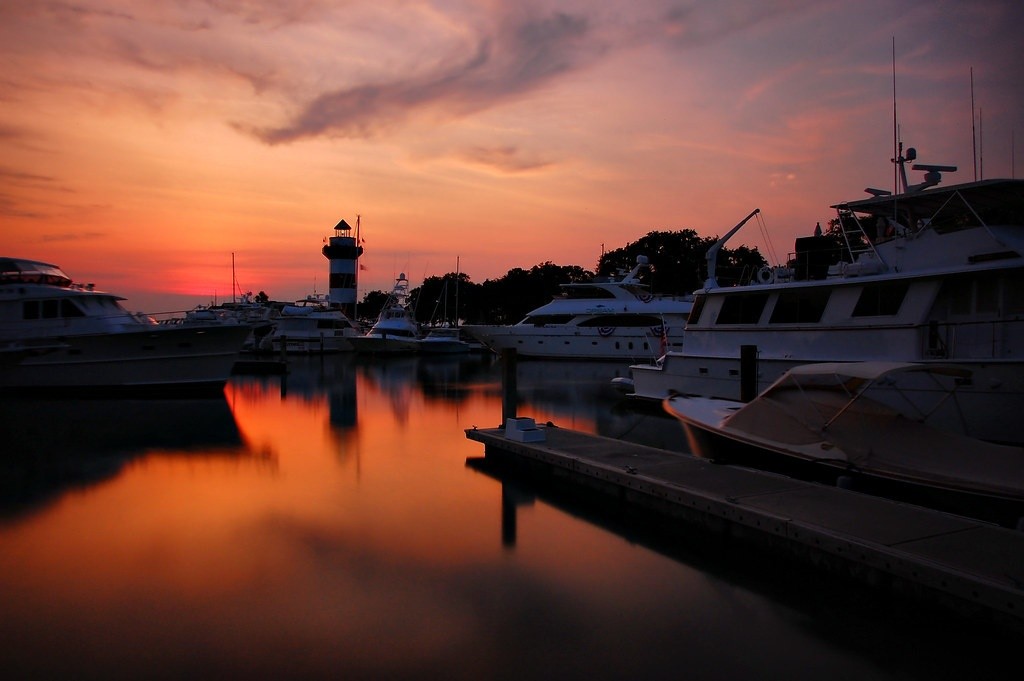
[184,149,1024,528]
[0,252,251,391]
